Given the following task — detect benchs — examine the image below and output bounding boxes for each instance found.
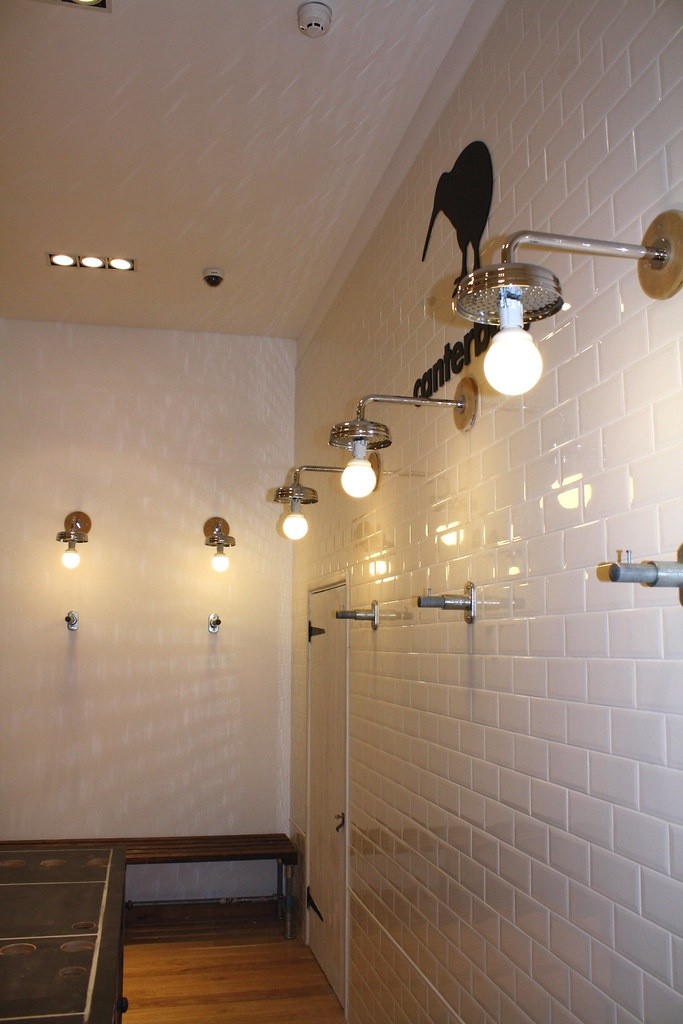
[0,833,298,938]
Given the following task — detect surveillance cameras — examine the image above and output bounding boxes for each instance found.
[203,268,224,287]
